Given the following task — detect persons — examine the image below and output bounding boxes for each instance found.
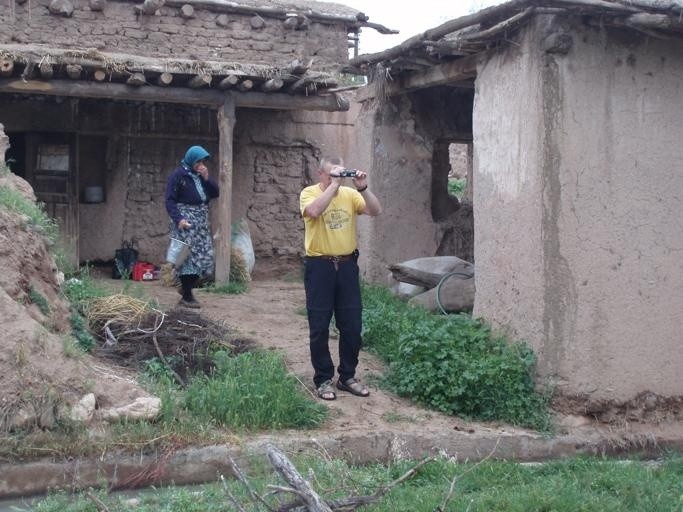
[298,155,384,402]
[165,146,219,309]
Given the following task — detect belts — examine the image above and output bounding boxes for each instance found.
[321,255,351,262]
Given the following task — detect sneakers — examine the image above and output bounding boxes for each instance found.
[179,296,201,308]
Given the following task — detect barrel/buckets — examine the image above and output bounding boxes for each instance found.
[164,230,194,269]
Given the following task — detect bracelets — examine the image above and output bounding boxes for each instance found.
[357,186,368,192]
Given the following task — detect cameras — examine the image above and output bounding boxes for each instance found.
[340,170,356,177]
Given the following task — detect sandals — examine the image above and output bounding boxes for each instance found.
[336,377,370,397]
[318,379,336,400]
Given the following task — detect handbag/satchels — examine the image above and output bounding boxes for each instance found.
[113,237,138,279]
[133,260,155,281]
